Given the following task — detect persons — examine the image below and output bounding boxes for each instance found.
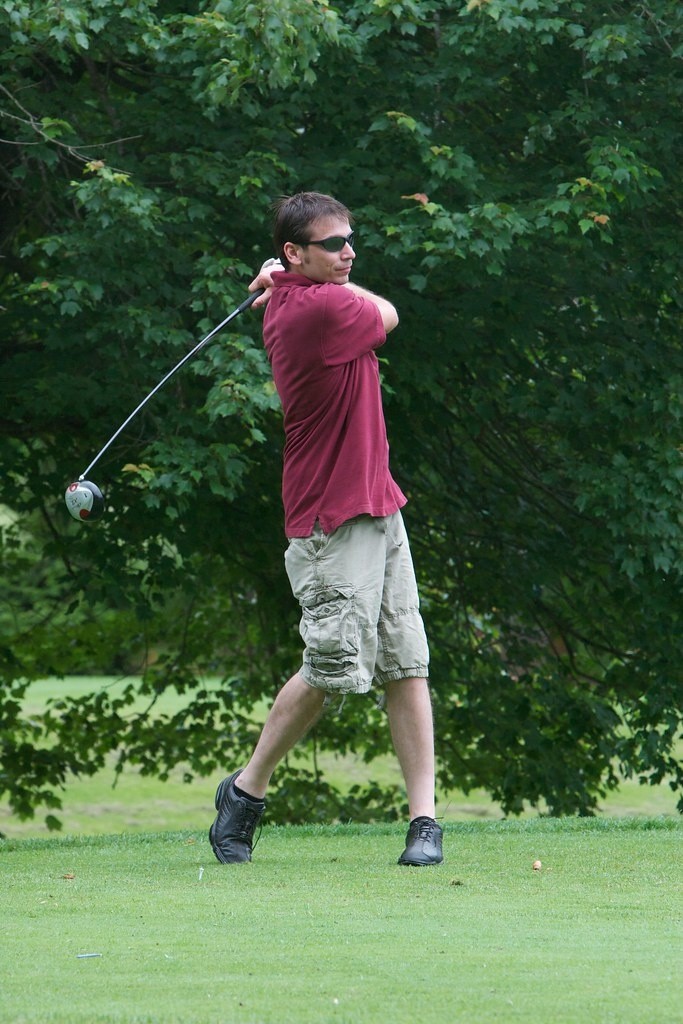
[210,191,445,865]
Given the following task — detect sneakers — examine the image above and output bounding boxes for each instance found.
[398,817,442,867]
[209,770,268,866]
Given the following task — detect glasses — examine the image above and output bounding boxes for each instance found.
[295,230,355,253]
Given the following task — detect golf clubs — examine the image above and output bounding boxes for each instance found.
[63,287,267,525]
